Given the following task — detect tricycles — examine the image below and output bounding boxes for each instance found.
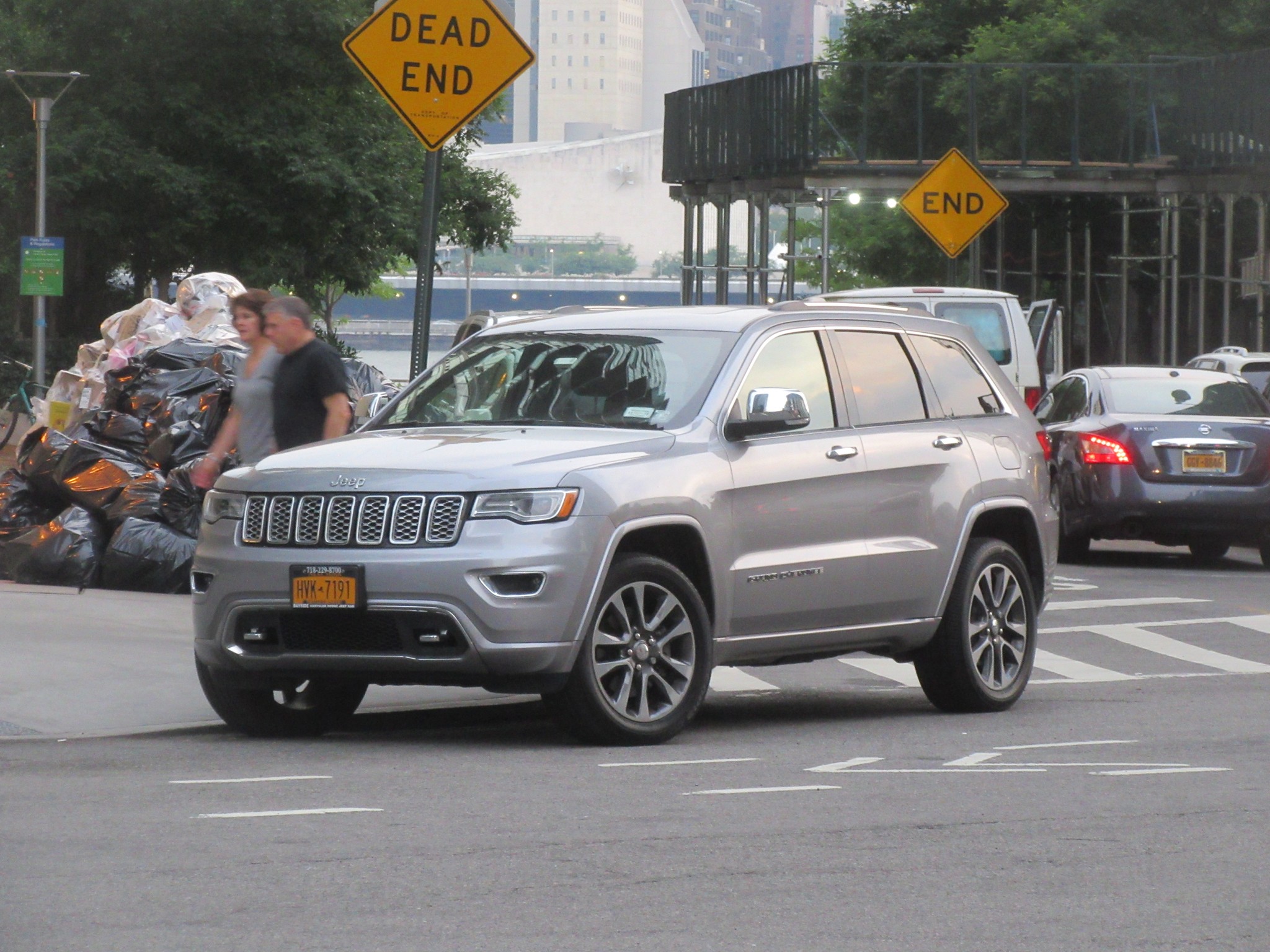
[0,354,55,450]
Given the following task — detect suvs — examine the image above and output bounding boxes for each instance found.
[186,299,1062,750]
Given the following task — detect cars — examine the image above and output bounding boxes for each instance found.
[450,302,648,353]
[804,285,1270,579]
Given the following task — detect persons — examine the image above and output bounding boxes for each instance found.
[190,289,284,488]
[261,297,354,453]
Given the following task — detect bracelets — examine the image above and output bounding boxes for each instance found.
[204,453,224,467]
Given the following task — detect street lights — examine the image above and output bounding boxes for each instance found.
[5,68,92,401]
[550,249,554,279]
